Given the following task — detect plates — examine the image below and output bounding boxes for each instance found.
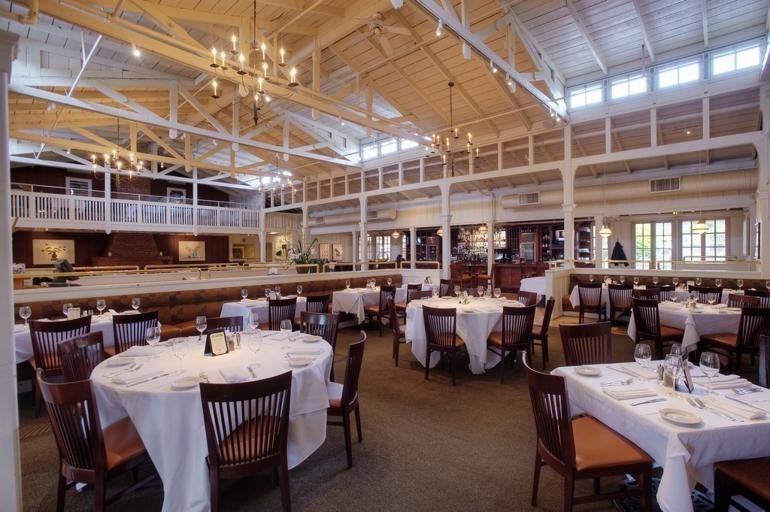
[105,357,135,366]
[573,365,601,376]
[659,408,702,427]
[170,375,199,390]
[300,336,323,343]
[286,357,314,367]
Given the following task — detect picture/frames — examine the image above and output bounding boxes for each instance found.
[31,238,76,265]
[178,240,206,262]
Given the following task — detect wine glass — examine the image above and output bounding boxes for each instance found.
[240,288,248,304]
[669,290,681,311]
[765,281,770,293]
[714,278,722,291]
[618,275,626,288]
[145,327,161,359]
[344,280,352,291]
[279,320,292,344]
[18,306,32,329]
[296,285,304,298]
[698,351,721,396]
[633,276,640,288]
[386,278,393,287]
[453,286,461,299]
[193,315,208,344]
[706,292,717,313]
[652,276,659,289]
[247,312,260,338]
[366,278,376,290]
[493,287,501,301]
[736,279,744,293]
[670,343,689,367]
[131,297,141,315]
[694,276,703,290]
[476,285,484,300]
[633,343,652,383]
[96,300,106,318]
[62,302,74,321]
[264,287,271,302]
[672,276,680,291]
[430,287,440,300]
[687,292,698,312]
[275,286,282,300]
[664,354,683,390]
[603,275,610,286]
[589,274,596,285]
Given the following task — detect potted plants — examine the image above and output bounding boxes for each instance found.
[40,243,67,261]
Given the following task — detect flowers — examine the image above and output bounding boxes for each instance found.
[283,237,321,271]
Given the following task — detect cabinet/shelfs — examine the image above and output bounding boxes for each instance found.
[399,215,595,292]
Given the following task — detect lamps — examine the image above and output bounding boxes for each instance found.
[258,156,297,199]
[390,1,516,96]
[208,0,301,128]
[692,218,710,235]
[391,232,400,240]
[421,79,480,178]
[599,225,614,238]
[90,117,143,184]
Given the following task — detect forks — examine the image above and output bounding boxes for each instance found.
[686,397,745,424]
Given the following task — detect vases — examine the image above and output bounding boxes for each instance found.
[296,267,310,274]
